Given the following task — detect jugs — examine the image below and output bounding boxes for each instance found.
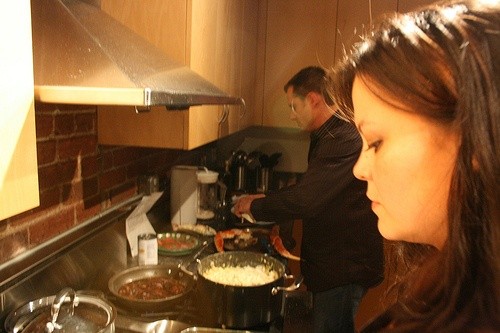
[195,171,226,219]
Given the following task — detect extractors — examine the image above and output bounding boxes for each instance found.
[30,0,246,114]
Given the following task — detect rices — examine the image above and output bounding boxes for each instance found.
[203,257,278,286]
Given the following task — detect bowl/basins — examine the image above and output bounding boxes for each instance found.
[108,260,198,311]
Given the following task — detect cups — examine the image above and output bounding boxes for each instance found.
[256,167,272,193]
[137,233,158,266]
[234,165,247,191]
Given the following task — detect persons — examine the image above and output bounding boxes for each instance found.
[324,1,498,333]
[229,65,384,333]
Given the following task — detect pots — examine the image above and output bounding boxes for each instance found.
[4,289,116,333]
[176,251,303,328]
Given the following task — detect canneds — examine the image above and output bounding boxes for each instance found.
[137,233,158,266]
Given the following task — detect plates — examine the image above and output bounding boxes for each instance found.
[222,235,296,256]
[157,231,205,257]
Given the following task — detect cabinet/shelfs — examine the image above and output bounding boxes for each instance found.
[99,0,440,149]
[0,0,40,221]
